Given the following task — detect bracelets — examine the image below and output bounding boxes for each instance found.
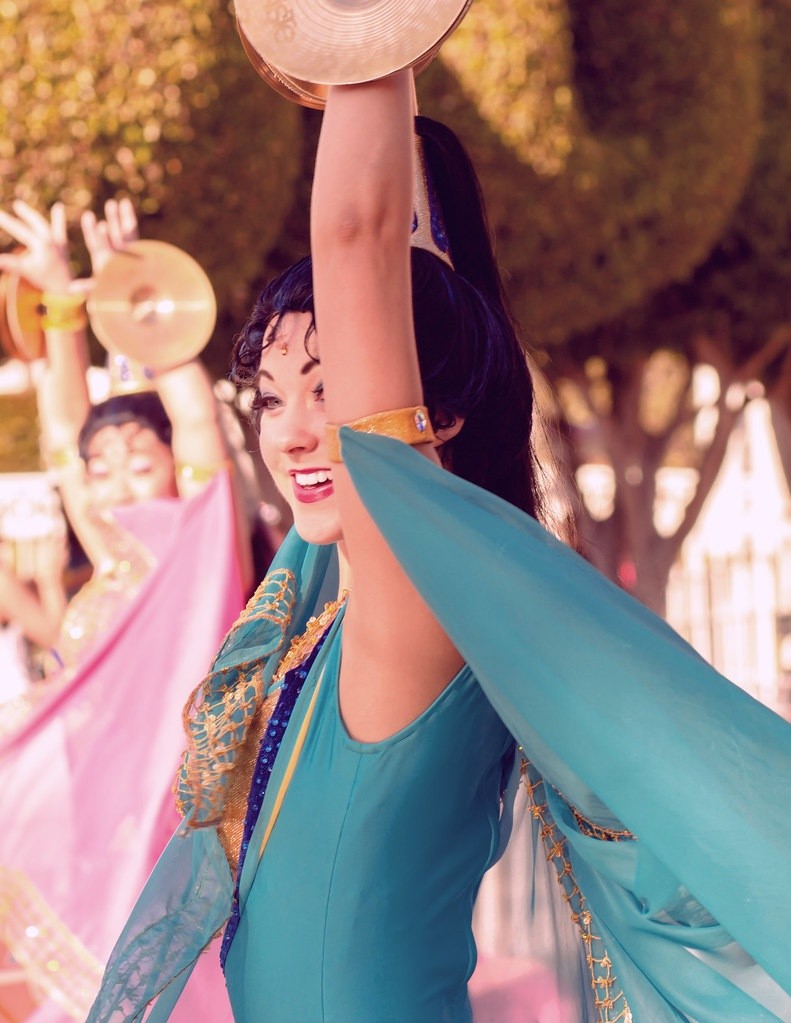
[38,291,88,330]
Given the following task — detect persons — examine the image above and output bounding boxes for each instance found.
[0,489,67,699]
[82,59,791,1023]
[0,198,575,1023]
